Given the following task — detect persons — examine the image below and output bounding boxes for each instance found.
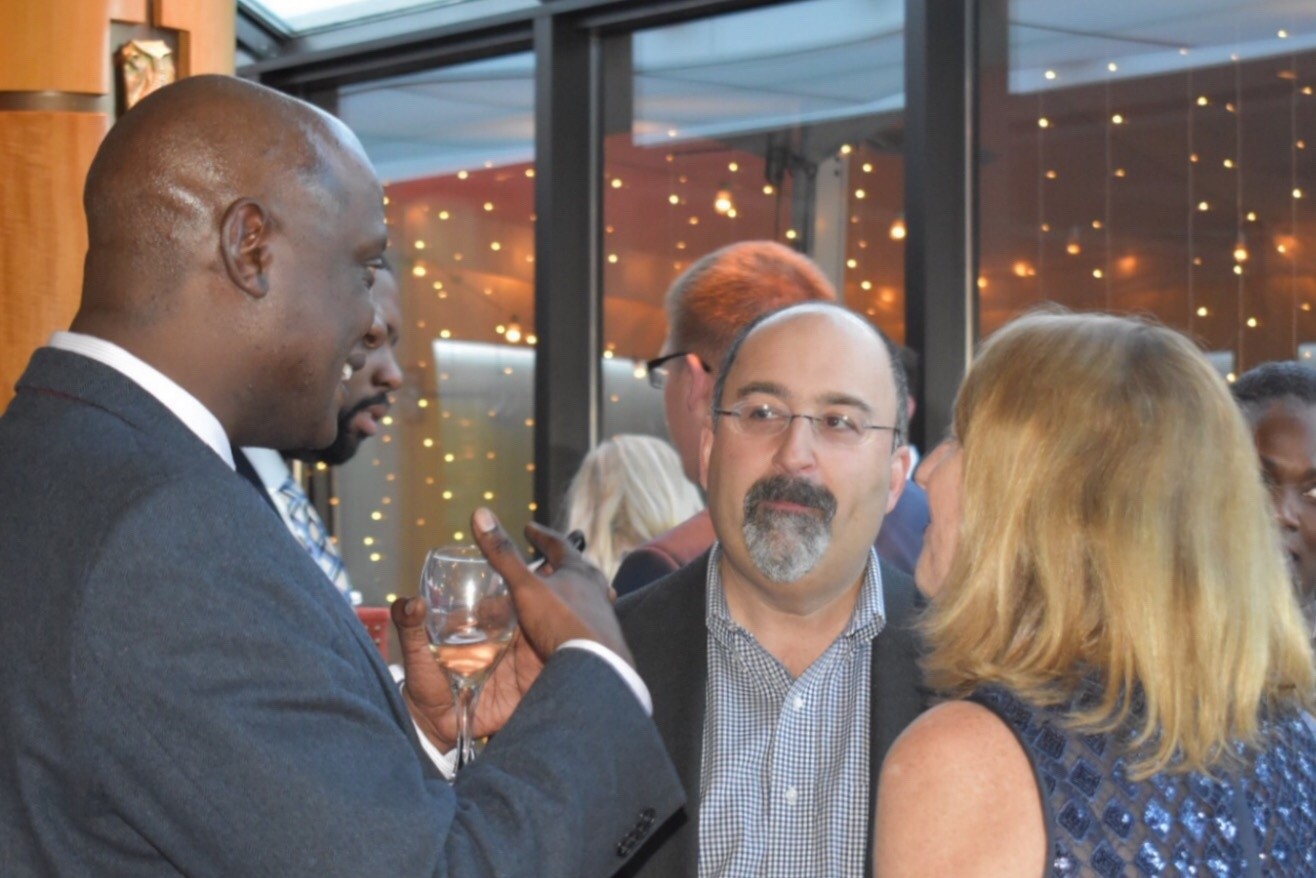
[613,299,949,878]
[0,75,686,878]
[872,311,1316,878]
[566,240,932,607]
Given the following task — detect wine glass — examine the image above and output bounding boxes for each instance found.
[417,546,520,784]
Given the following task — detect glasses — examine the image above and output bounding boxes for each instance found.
[647,352,712,390]
[714,401,900,445]
